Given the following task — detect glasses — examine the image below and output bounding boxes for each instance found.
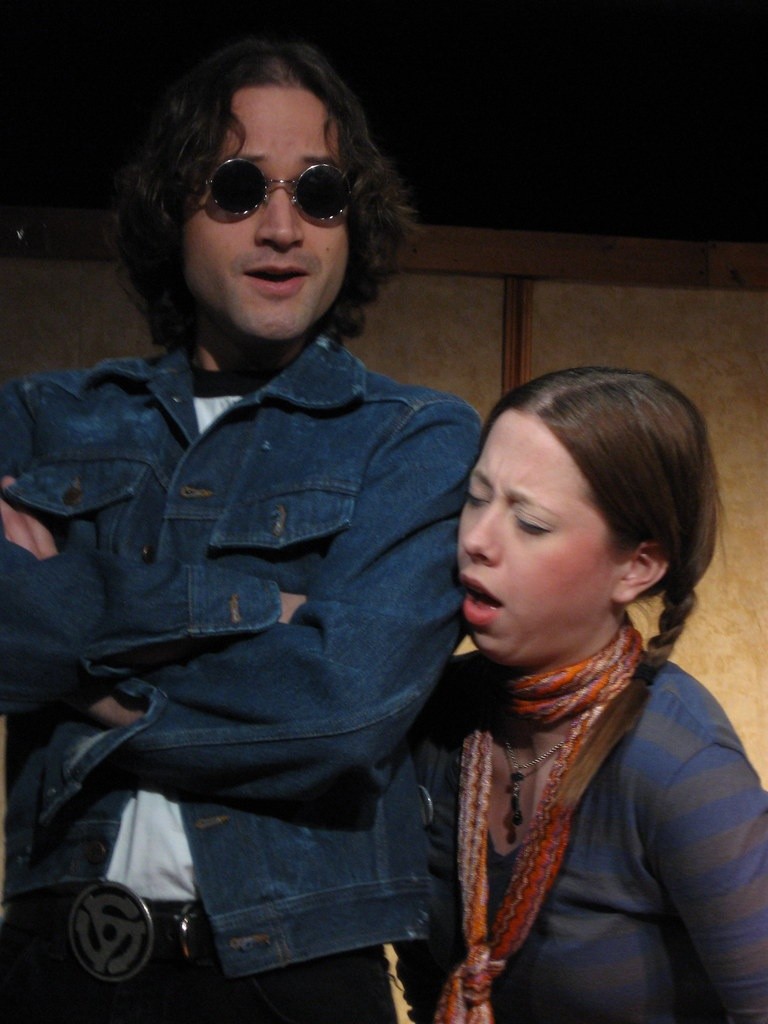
[205,157,352,222]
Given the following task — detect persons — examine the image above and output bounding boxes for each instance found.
[0,25,486,1024]
[361,368,768,1024]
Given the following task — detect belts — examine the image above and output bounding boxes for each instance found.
[3,880,214,983]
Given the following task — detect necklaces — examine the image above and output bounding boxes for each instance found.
[500,714,568,826]
